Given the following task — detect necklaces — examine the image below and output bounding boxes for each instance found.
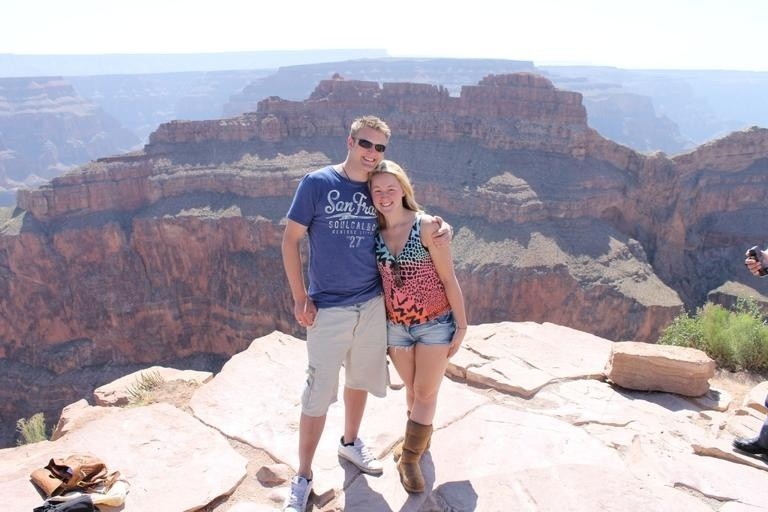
[340,163,352,184]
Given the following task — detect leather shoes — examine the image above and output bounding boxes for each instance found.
[733,437,768,460]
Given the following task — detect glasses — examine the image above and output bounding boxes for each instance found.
[388,259,403,288]
[351,137,386,153]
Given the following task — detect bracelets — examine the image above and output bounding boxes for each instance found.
[457,326,467,330]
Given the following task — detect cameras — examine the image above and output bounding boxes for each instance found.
[749,246,768,277]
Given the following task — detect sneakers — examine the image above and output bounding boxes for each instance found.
[281,468,313,512]
[336,436,384,474]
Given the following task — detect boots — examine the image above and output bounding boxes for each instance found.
[393,409,433,493]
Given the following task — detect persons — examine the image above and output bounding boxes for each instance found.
[731,246,768,464]
[366,158,468,496]
[281,112,455,512]
